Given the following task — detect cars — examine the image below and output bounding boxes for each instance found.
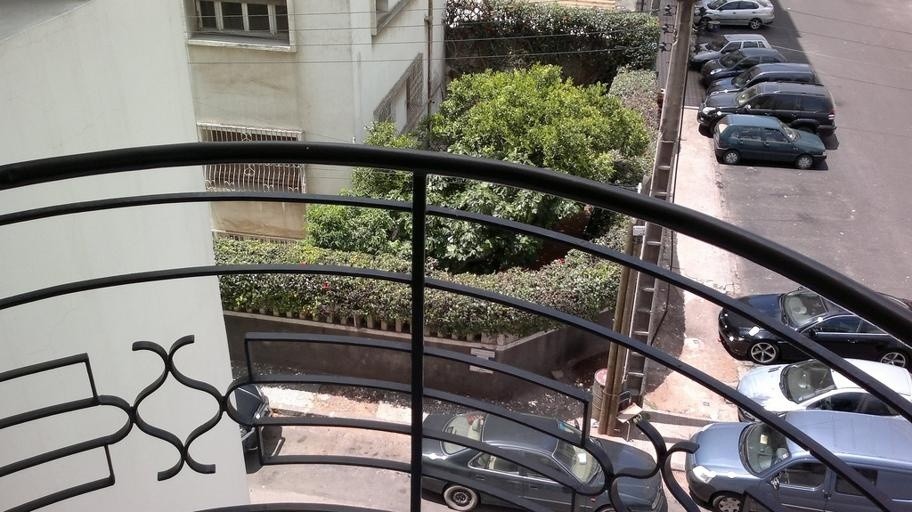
[413,410,668,511]
[733,356,912,425]
[232,375,271,448]
[718,287,911,371]
[690,0,839,171]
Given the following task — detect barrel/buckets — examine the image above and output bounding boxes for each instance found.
[590,368,608,422]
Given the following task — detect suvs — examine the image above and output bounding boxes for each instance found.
[676,408,912,511]
